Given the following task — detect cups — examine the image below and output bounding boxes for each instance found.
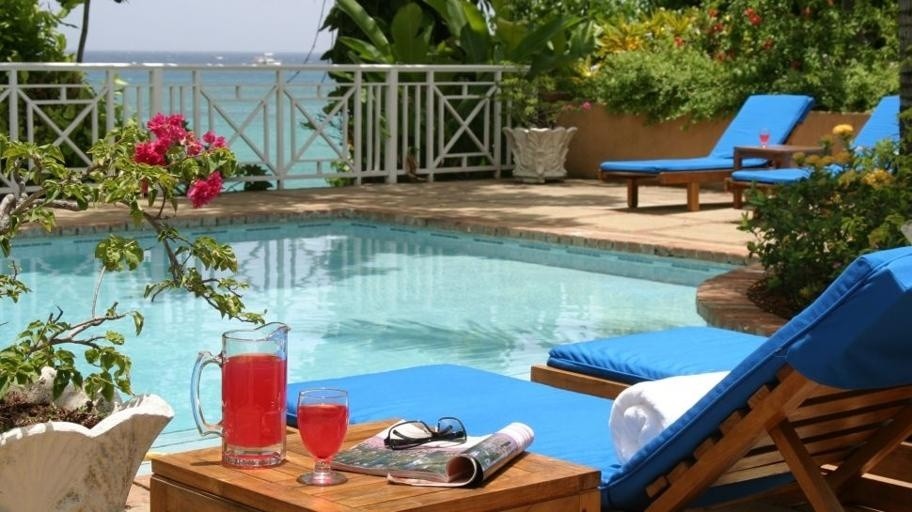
[189,321,291,467]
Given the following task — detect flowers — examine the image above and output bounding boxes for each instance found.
[493,62,599,126]
[0,111,264,408]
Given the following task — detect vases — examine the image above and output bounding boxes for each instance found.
[0,366,173,511]
[504,126,579,183]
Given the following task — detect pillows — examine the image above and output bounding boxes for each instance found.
[612,370,732,463]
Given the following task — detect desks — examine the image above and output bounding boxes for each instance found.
[733,145,824,212]
[147,418,600,512]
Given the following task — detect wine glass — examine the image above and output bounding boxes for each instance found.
[295,390,349,487]
[758,129,771,149]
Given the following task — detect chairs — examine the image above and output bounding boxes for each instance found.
[531,323,768,399]
[601,93,814,213]
[726,94,901,211]
[286,245,912,512]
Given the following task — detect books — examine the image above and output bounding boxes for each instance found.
[331,419,526,487]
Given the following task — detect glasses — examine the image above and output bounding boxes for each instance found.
[384,416,466,450]
